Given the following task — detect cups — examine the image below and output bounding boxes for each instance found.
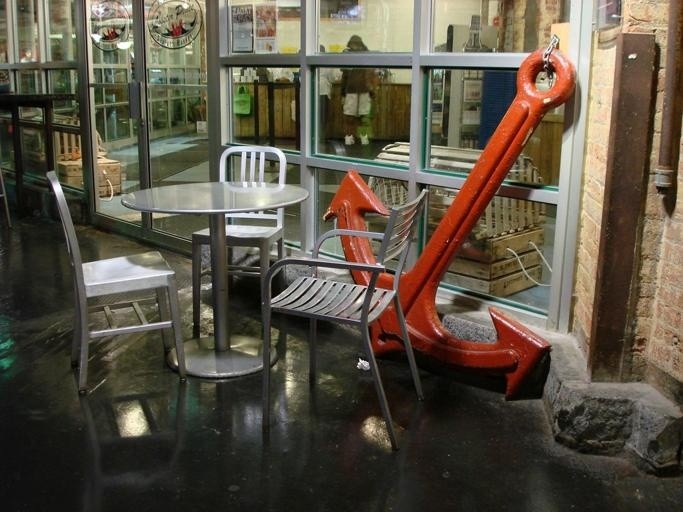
[234,75,253,82]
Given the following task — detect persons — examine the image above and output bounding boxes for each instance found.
[338,34,376,147]
[316,43,343,145]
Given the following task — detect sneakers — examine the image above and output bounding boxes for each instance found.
[359,132,369,144]
[343,134,355,145]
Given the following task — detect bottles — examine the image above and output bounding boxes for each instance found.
[461,15,482,52]
